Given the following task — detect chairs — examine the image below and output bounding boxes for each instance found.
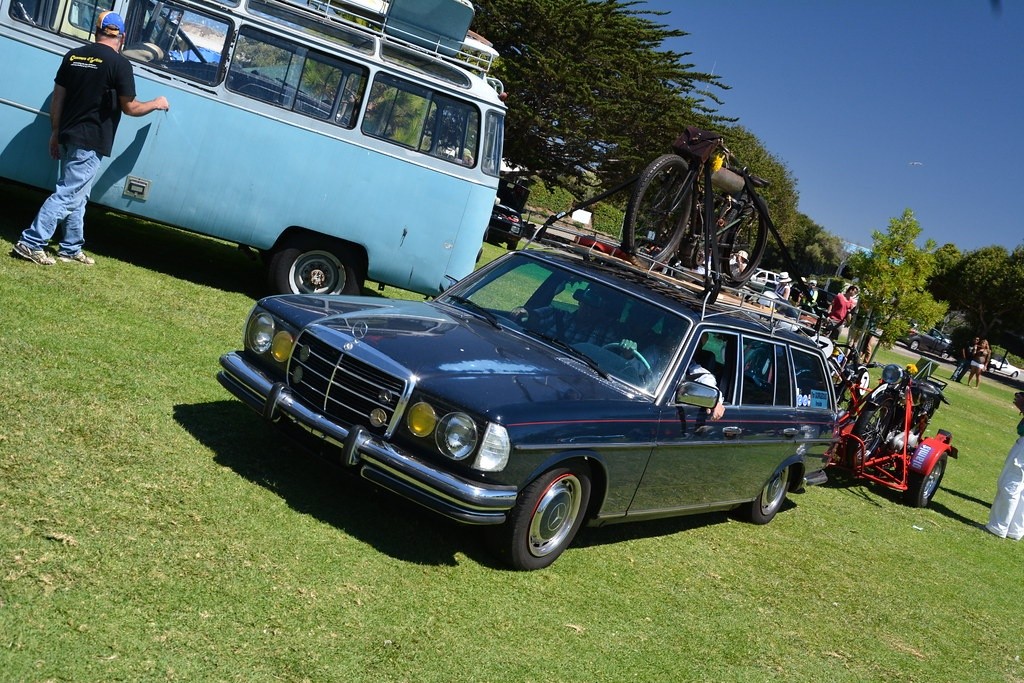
[598,290,626,329]
[699,350,716,371]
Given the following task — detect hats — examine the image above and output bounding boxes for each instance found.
[800,276,807,283]
[735,250,749,262]
[808,279,818,287]
[776,271,792,283]
[96,10,124,36]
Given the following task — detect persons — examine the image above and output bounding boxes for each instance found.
[694,245,749,277]
[983,391,1024,540]
[510,283,725,422]
[13,11,169,266]
[776,272,818,310]
[827,286,878,366]
[950,337,991,389]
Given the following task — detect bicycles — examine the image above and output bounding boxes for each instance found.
[622,126,788,296]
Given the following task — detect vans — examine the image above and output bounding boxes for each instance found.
[0,0,510,295]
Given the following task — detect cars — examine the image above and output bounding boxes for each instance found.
[896,324,952,360]
[211,235,839,572]
[986,353,1020,378]
[750,266,878,334]
[483,206,526,252]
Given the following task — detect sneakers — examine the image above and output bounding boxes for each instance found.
[12,242,56,266]
[56,249,95,265]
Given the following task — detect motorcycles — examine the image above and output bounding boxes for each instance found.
[846,358,953,469]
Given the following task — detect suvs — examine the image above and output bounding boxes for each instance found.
[159,60,351,124]
